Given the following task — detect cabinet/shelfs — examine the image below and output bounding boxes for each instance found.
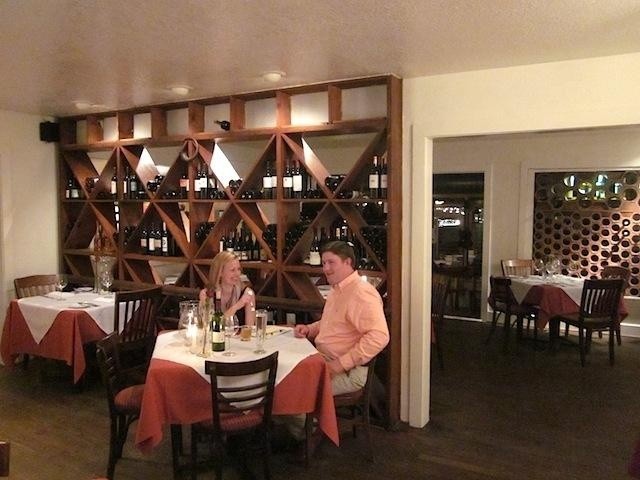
[56,73,403,429]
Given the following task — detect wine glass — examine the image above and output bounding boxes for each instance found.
[55,279,69,301]
[218,314,240,357]
[252,309,267,354]
[101,273,114,298]
[532,258,562,285]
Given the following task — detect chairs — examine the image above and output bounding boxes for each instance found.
[590,265,631,344]
[486,276,538,354]
[78,286,162,392]
[550,278,625,367]
[288,354,374,467]
[13,275,60,370]
[95,332,183,480]
[495,259,544,335]
[192,351,279,480]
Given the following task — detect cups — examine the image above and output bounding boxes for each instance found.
[240,325,252,341]
[88,254,117,294]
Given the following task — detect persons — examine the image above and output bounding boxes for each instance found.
[199,252,257,329]
[282,241,390,461]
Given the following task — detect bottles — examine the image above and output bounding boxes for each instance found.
[209,288,227,351]
[179,299,212,361]
[64,154,387,271]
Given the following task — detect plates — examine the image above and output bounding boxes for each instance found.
[74,287,93,292]
[69,303,91,308]
